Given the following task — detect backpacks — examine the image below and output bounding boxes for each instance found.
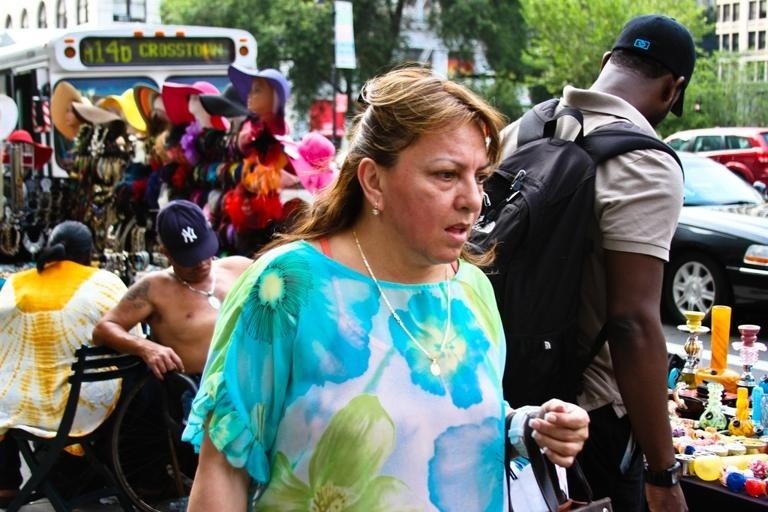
[462,99,683,410]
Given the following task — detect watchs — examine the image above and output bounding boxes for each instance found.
[639,460,685,490]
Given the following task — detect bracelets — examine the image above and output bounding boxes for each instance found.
[507,404,533,459]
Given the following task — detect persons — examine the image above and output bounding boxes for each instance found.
[93,199,256,481]
[175,63,590,512]
[41,65,298,247]
[0,218,142,512]
[460,14,697,511]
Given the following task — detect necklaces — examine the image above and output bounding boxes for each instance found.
[166,266,223,310]
[349,228,451,377]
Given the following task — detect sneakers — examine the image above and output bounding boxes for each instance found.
[1,489,21,510]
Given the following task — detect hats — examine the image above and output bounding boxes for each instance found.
[3,132,52,170]
[614,16,693,117]
[276,132,337,193]
[155,200,219,268]
[50,66,289,141]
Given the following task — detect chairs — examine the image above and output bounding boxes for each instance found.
[6,342,143,511]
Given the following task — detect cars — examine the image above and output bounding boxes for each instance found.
[649,123,768,340]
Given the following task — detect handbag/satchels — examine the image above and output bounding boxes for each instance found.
[530,497,612,511]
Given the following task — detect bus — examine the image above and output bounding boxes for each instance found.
[0,19,259,183]
